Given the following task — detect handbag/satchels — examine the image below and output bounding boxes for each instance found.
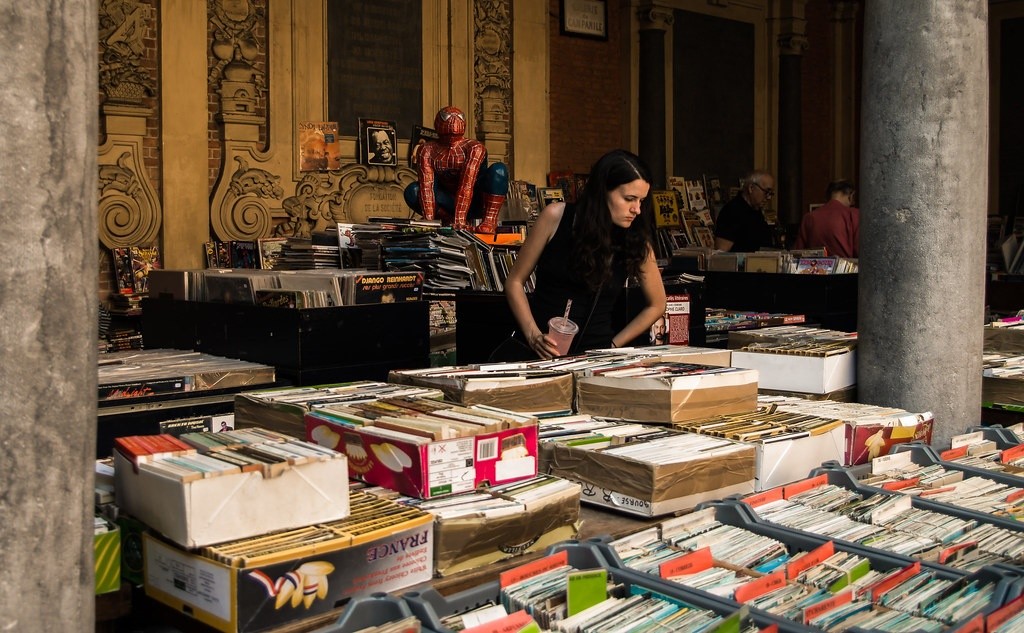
[488,338,541,363]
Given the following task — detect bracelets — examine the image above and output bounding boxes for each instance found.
[611,341,617,348]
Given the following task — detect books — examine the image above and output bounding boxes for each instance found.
[93,116,1024,633]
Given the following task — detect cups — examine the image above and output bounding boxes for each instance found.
[547,317,578,359]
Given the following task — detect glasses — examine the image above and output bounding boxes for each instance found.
[755,182,775,197]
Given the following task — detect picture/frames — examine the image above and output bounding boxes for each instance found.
[558,0,609,42]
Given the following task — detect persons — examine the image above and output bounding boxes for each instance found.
[714,169,777,253]
[795,179,860,258]
[369,130,394,164]
[651,315,667,346]
[217,421,233,433]
[505,148,667,361]
[403,106,509,235]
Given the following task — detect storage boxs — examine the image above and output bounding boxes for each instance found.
[984,326,1024,353]
[982,375,1024,406]
[731,348,856,395]
[386,366,575,414]
[647,343,733,367]
[661,352,731,367]
[304,404,541,498]
[110,422,353,553]
[540,427,758,519]
[845,419,934,468]
[432,472,581,579]
[734,421,846,493]
[92,521,124,596]
[234,382,445,445]
[144,492,437,633]
[578,367,760,422]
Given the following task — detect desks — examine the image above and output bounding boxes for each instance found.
[687,270,859,333]
[136,278,705,389]
[981,401,1024,412]
